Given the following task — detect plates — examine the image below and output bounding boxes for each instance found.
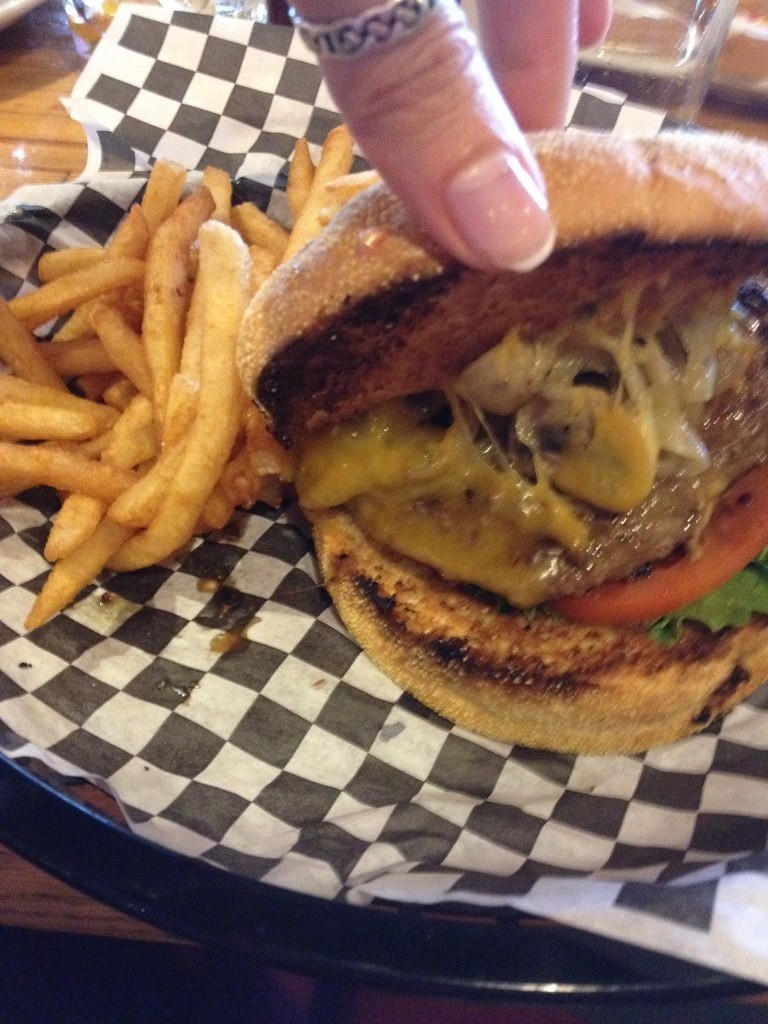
[0,74,768,1002]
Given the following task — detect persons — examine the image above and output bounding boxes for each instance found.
[293,0,612,280]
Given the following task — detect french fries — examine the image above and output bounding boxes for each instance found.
[0,123,383,631]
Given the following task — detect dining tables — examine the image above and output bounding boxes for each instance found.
[0,2,766,1017]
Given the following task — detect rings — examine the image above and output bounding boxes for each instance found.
[292,0,449,62]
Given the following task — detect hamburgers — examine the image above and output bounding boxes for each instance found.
[233,125,768,756]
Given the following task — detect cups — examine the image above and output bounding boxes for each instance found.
[595,0,727,128]
